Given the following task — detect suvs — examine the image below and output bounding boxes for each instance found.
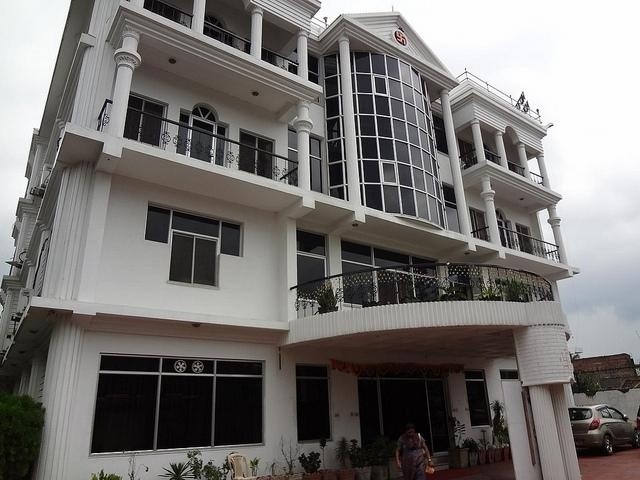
[569,405,640,455]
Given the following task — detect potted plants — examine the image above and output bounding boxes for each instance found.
[445,413,468,469]
[337,437,355,480]
[250,458,260,476]
[504,427,510,461]
[297,450,321,480]
[370,434,388,480]
[490,399,504,463]
[314,281,340,314]
[389,442,405,479]
[464,436,478,466]
[319,428,337,480]
[350,443,373,479]
